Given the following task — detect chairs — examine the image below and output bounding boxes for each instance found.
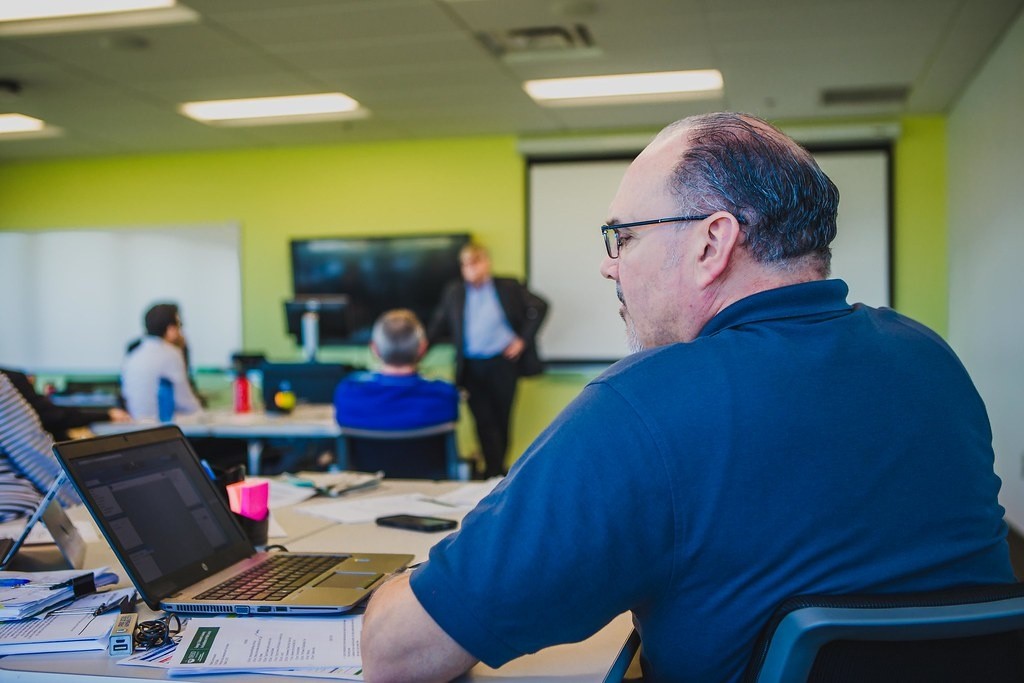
[341,422,456,481]
[744,583,1024,683]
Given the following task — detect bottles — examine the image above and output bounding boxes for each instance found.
[157,376,175,422]
[234,372,250,413]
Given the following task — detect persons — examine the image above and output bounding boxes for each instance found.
[0,375,83,524]
[360,111,1024,683]
[120,303,205,421]
[332,311,461,479]
[433,241,550,479]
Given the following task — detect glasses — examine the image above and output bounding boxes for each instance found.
[600,212,749,259]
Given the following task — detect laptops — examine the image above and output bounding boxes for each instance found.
[53,423,415,616]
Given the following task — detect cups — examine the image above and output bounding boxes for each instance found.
[211,465,245,507]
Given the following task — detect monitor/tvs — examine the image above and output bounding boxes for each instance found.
[285,297,351,365]
[290,233,471,348]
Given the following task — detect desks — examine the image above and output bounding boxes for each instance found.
[0,471,636,683]
[67,404,341,474]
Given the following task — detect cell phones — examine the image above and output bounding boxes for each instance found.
[377,515,458,532]
